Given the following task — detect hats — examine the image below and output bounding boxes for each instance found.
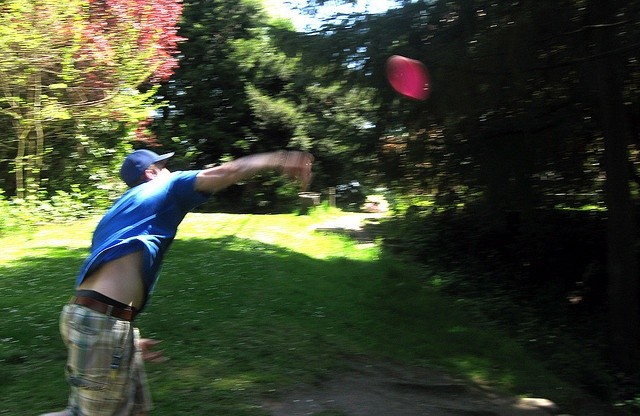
[120,148,176,187]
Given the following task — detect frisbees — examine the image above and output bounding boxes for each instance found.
[388,56,432,101]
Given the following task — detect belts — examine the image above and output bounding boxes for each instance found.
[72,296,134,321]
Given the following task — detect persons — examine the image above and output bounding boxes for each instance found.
[42,149,314,415]
